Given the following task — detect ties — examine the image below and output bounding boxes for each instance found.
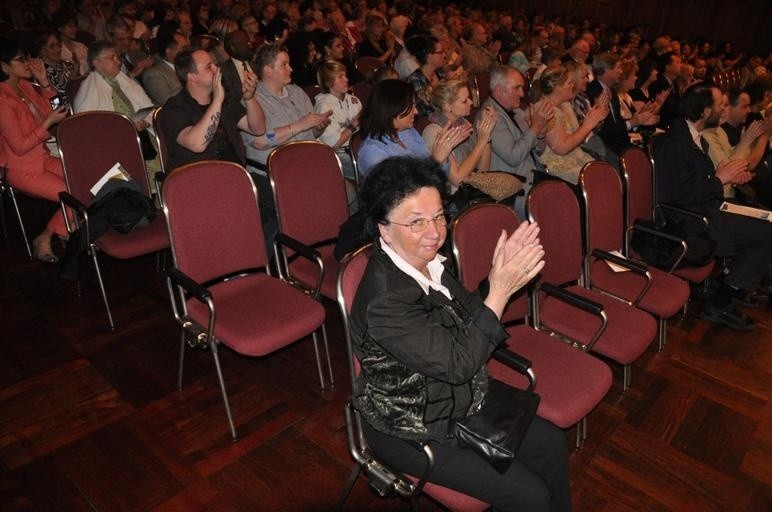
[121,52,151,99]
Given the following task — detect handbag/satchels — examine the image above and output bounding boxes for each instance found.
[354,58,386,79]
[453,378,541,475]
[464,170,524,201]
[631,219,715,271]
[393,48,420,78]
[450,183,515,209]
[9,56,27,62]
[44,136,61,158]
[333,207,373,263]
[137,129,156,161]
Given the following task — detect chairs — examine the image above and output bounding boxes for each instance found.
[578,159,690,387]
[153,106,170,175]
[266,139,351,386]
[157,159,327,440]
[354,56,388,77]
[524,179,660,441]
[54,110,170,329]
[302,84,326,98]
[649,130,721,312]
[472,50,537,107]
[338,242,537,511]
[450,204,614,456]
[621,148,716,345]
[350,129,367,193]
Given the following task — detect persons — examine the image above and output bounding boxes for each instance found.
[346,153,573,512]
[352,0,772,332]
[0,0,363,262]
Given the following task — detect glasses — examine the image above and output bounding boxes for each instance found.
[378,214,450,232]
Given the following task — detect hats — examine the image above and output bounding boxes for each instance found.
[508,51,531,74]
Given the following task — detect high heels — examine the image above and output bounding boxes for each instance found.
[32,238,60,263]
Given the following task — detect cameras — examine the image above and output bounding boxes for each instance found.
[49,94,68,113]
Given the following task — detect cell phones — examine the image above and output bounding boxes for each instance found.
[242,60,250,80]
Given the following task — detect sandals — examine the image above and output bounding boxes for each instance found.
[702,294,755,330]
[710,277,761,308]
